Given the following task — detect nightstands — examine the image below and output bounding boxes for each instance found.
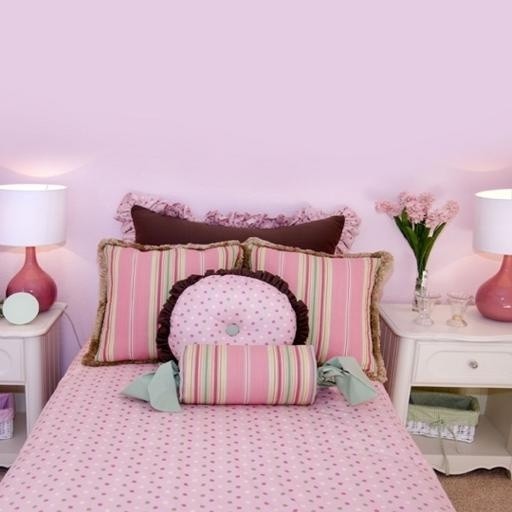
[1,301,67,469]
[373,303,511,480]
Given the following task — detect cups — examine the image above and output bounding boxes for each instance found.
[414,288,474,328]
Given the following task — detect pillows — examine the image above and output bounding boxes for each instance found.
[154,270,311,368]
[242,238,395,385]
[118,343,382,416]
[112,193,363,254]
[82,239,248,366]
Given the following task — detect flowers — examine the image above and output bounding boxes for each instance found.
[374,190,462,291]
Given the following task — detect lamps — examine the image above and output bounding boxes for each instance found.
[475,189,512,323]
[0,183,67,315]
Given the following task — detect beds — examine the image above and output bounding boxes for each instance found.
[4,332,455,511]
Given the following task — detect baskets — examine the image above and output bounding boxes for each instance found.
[0,392,16,440]
[404,390,480,443]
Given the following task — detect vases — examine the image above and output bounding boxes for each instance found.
[411,278,429,312]
